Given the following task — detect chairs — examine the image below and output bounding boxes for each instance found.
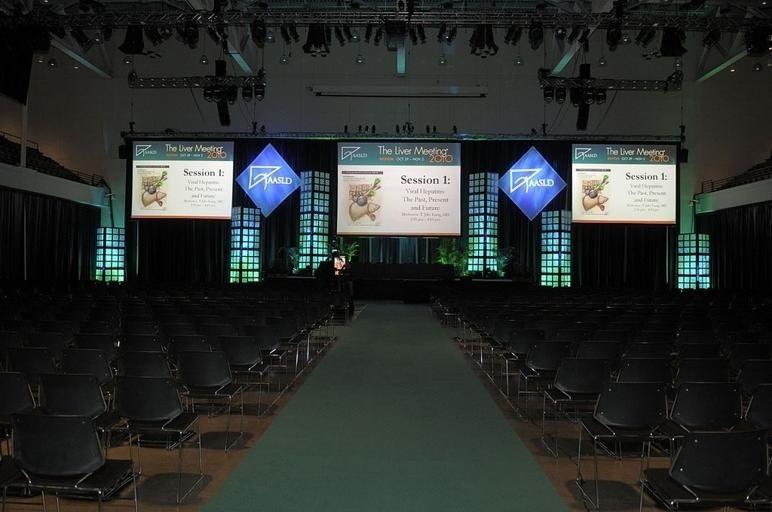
[1,283,355,512]
[430,285,770,511]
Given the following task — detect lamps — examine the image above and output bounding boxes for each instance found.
[3,3,771,75]
[202,84,265,104]
[537,68,550,88]
[543,87,606,105]
